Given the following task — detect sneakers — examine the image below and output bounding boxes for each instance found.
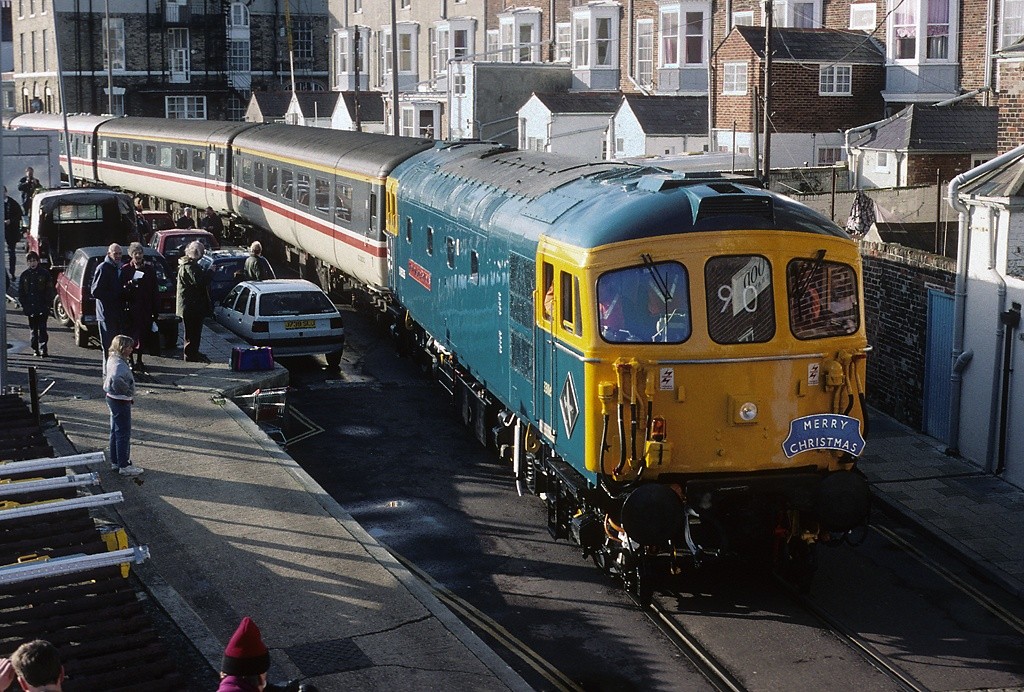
[111,463,119,469]
[120,465,144,476]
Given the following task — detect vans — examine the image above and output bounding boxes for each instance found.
[22,188,141,298]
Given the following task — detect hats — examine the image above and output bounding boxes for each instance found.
[250,241,263,252]
[220,615,271,676]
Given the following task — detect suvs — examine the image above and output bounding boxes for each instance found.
[52,246,182,350]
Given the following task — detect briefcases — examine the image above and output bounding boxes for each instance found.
[231,346,274,371]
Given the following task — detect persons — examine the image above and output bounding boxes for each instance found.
[0,638,65,692]
[101,335,144,475]
[596,274,678,340]
[216,617,270,692]
[2,167,268,382]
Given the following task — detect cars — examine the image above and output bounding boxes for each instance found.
[213,277,345,367]
[138,210,175,247]
[197,246,277,309]
[146,228,221,278]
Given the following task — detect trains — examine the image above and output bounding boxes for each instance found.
[0,108,876,605]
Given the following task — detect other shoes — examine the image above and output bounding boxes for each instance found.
[184,351,208,363]
[129,362,145,372]
[34,349,48,357]
